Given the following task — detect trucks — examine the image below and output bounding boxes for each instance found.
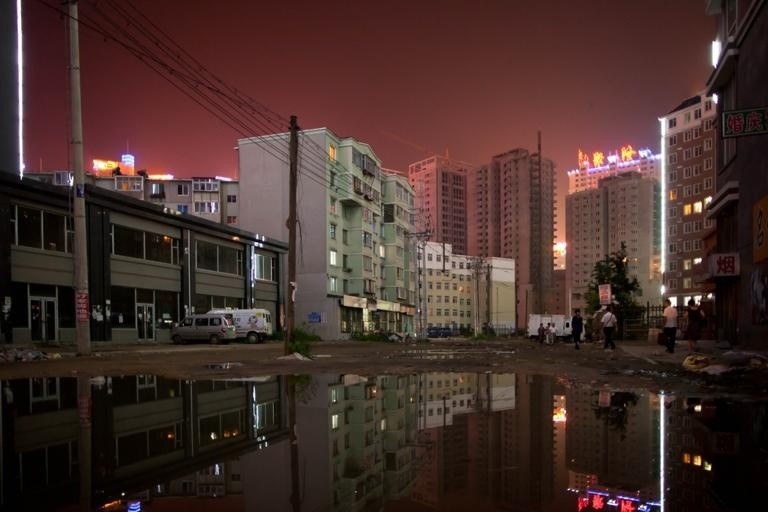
[525,313,586,343]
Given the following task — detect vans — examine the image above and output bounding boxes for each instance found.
[169,307,272,345]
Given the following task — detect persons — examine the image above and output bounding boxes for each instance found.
[571,309,584,351]
[684,299,707,351]
[661,299,679,353]
[538,322,561,346]
[597,305,619,350]
